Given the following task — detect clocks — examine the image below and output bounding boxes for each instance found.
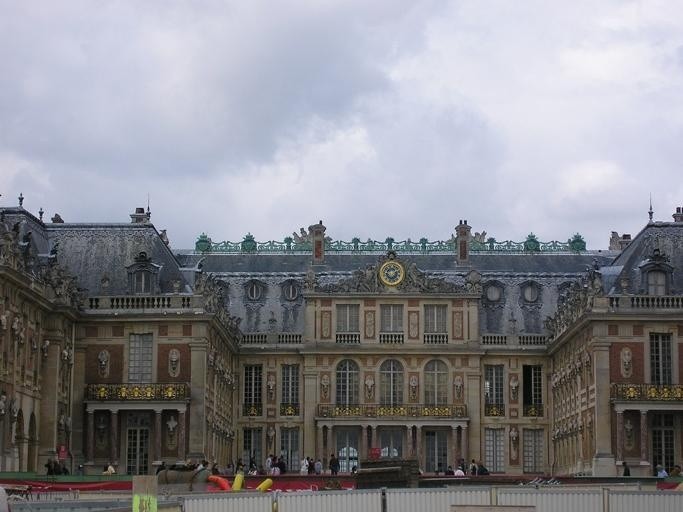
[378,261,404,287]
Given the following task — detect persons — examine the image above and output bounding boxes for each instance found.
[312,458,322,474]
[29,331,40,352]
[266,426,275,447]
[408,262,430,293]
[41,258,90,313]
[328,453,337,476]
[432,470,441,477]
[477,464,489,475]
[670,465,683,477]
[211,463,220,475]
[304,456,308,466]
[469,458,478,475]
[357,265,377,294]
[171,350,178,370]
[0,395,7,415]
[508,427,519,444]
[269,456,280,475]
[10,315,18,335]
[105,462,115,476]
[276,456,286,473]
[445,465,454,475]
[655,464,668,477]
[509,374,518,392]
[622,460,630,475]
[235,465,244,474]
[234,458,246,473]
[196,271,245,348]
[0,310,12,330]
[539,257,603,335]
[197,459,205,471]
[307,457,314,474]
[17,327,25,345]
[453,465,465,476]
[60,345,70,362]
[154,460,165,474]
[247,463,257,475]
[351,465,357,472]
[98,350,107,366]
[263,453,273,474]
[40,339,51,360]
[223,464,233,475]
[199,460,208,471]
